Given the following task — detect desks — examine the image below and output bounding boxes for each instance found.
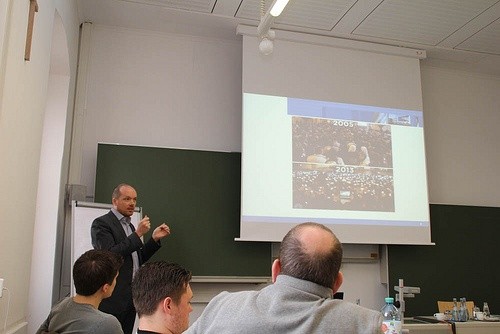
[400,316,500,334]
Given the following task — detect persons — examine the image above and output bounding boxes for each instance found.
[131,255,193,334]
[182,222,383,334]
[91,183,171,334]
[307,139,370,167]
[36,249,124,334]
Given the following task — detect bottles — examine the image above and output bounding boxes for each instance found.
[483,302,490,318]
[451,297,468,321]
[379,298,402,334]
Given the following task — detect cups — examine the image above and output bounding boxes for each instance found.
[434,313,444,321]
[475,311,487,320]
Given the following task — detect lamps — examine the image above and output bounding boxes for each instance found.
[258,0,290,37]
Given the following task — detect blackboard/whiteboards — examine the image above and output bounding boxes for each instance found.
[95,143,273,284]
[71,200,141,300]
[386,204,500,318]
[274,242,380,264]
